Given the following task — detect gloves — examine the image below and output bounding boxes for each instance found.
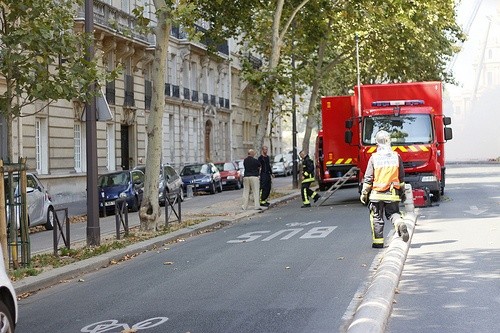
[360,183,372,204]
[400,182,406,203]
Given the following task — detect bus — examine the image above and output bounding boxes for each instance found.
[99,169,145,217]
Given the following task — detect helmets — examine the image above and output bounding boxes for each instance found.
[376,130,391,146]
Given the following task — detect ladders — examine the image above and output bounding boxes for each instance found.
[316,166,370,206]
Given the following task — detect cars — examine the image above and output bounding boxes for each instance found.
[270,147,315,178]
[179,162,222,195]
[208,158,245,190]
[0,241,19,333]
[4,171,55,234]
[130,164,184,207]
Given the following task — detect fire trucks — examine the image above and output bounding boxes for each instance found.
[313,39,452,208]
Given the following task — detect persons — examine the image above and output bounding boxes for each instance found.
[360,130,409,249]
[257,146,275,207]
[241,149,262,210]
[299,150,321,208]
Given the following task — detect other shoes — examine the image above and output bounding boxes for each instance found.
[260,202,270,206]
[372,243,384,248]
[399,223,409,242]
[301,204,311,208]
[314,193,321,203]
[257,208,262,210]
